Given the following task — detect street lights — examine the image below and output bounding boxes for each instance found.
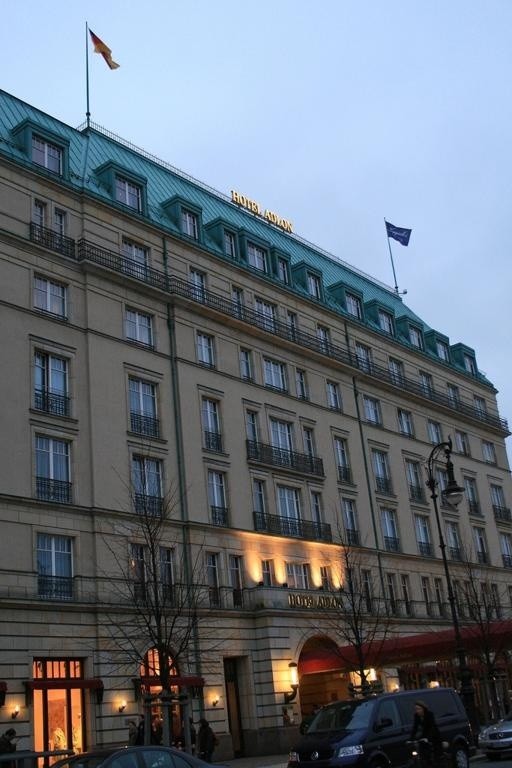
[427,442,479,738]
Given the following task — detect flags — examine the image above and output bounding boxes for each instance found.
[381,217,413,250]
[85,30,122,71]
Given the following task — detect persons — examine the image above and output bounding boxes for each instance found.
[150,715,164,745]
[128,718,139,746]
[135,712,145,744]
[181,718,196,754]
[1,728,18,767]
[198,719,216,762]
[408,702,443,767]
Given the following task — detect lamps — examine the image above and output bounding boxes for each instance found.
[213,695,219,707]
[119,698,127,712]
[12,704,20,719]
[284,662,299,703]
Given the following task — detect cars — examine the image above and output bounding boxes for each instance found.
[478,713,511,759]
[51,745,231,768]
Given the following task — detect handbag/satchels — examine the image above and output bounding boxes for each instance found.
[214,738,220,745]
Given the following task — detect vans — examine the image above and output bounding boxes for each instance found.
[288,686,476,768]
